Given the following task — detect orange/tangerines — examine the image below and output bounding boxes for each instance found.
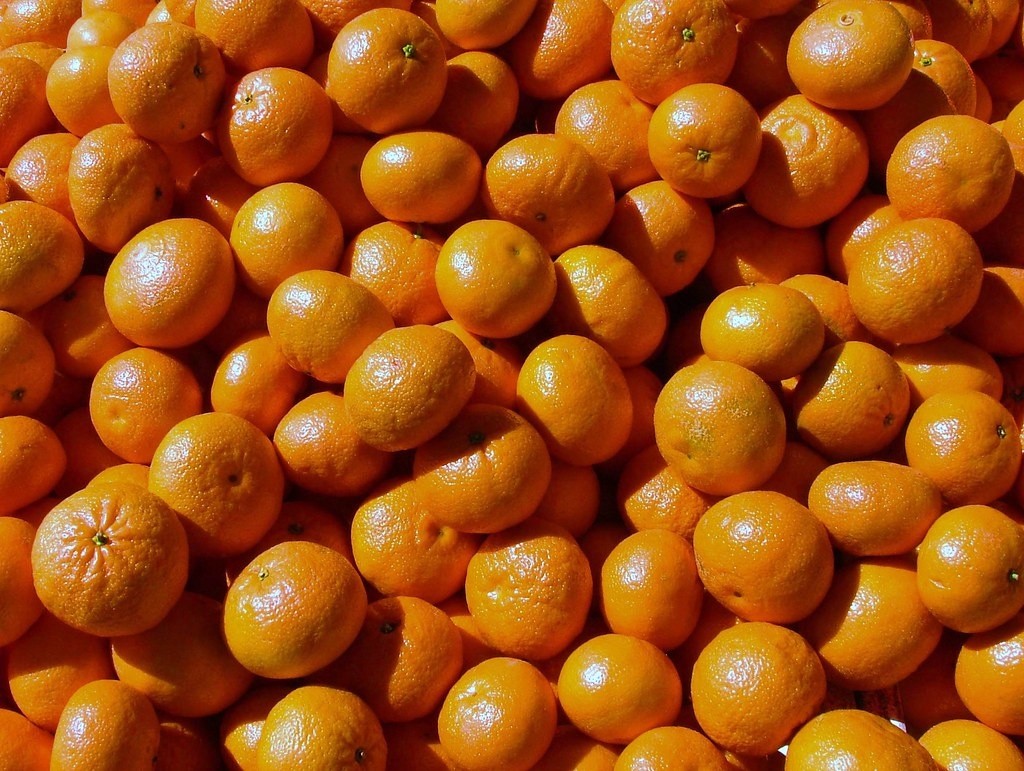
[0,0,1024,771]
